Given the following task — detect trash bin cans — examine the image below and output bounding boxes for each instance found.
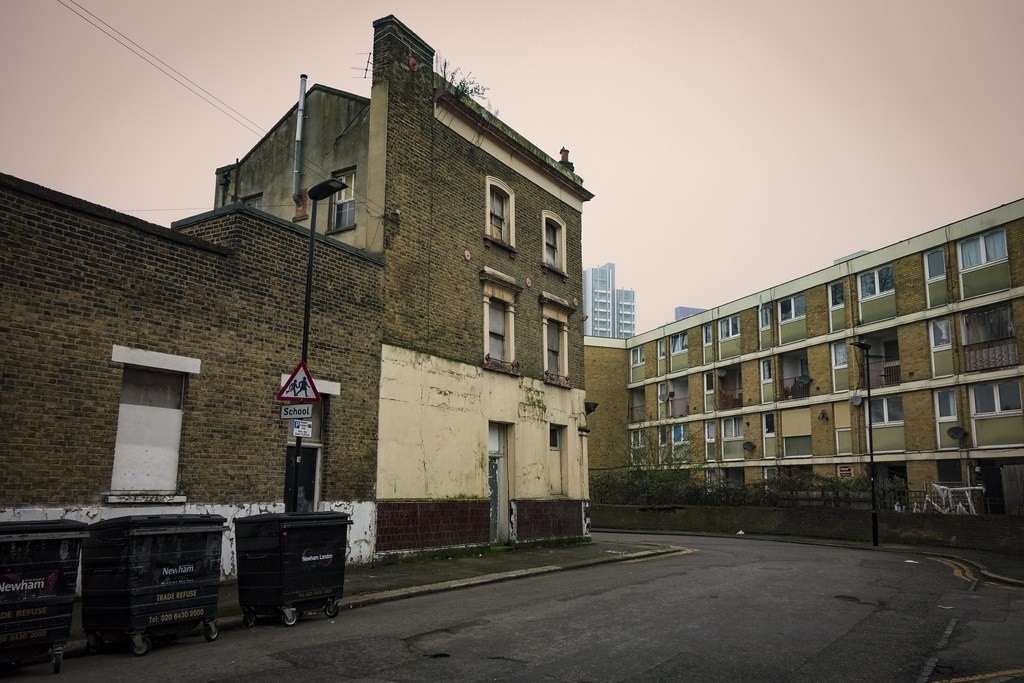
[80,514,232,656]
[0,519,87,676]
[232,511,355,628]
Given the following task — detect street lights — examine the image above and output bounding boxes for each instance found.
[849,341,880,545]
[276,179,349,513]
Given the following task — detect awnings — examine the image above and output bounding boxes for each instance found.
[585,401,600,415]
